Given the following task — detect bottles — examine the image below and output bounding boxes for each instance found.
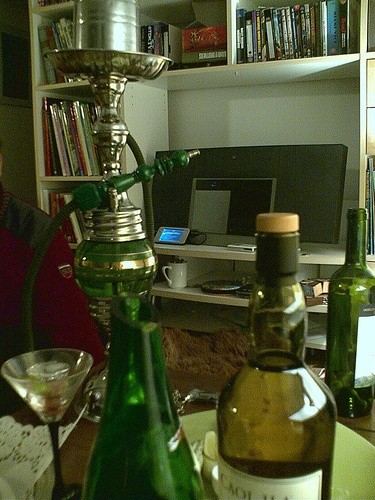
[81,292,208,500]
[325,208,375,418]
[216,212,338,500]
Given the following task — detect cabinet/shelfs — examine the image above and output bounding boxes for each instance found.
[29,0,375,350]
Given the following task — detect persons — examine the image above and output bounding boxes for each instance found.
[0,139,104,419]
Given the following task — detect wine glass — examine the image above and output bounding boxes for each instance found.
[0,347,94,499]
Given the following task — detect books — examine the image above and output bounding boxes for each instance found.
[37,17,82,84]
[365,155,375,256]
[140,22,227,70]
[236,0,361,64]
[43,97,105,177]
[49,191,100,245]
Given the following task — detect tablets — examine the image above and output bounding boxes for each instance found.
[154,226,191,244]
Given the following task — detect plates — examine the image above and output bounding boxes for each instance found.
[179,409,375,499]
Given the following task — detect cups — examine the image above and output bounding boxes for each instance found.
[161,260,188,289]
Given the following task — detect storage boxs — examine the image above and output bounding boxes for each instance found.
[300,278,331,305]
[141,21,227,71]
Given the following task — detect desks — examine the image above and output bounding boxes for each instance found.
[11,395,375,500]
